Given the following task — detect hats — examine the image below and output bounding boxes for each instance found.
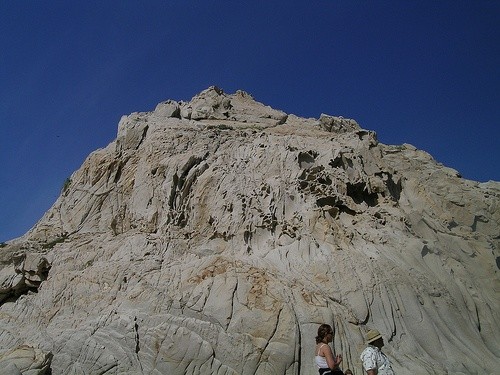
[364,329,382,343]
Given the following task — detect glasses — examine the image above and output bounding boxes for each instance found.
[328,331,333,335]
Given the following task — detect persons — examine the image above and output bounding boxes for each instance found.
[344,369,353,375]
[315,323,345,375]
[360,329,395,375]
